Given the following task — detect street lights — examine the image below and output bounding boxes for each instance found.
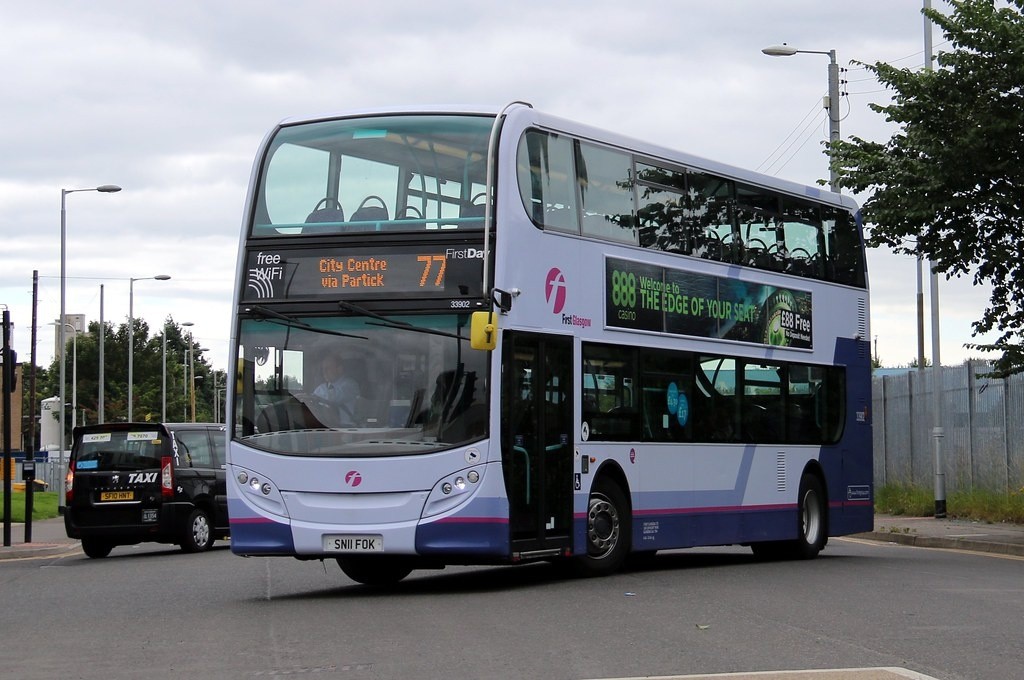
[58,185,124,516]
[47,322,77,448]
[184,348,210,424]
[127,275,172,422]
[761,43,841,193]
[212,369,226,423]
[162,321,195,421]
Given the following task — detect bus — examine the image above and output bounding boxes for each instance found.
[226,101,880,587]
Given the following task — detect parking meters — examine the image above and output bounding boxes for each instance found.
[22,459,38,542]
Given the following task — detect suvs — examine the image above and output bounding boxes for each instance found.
[63,420,230,558]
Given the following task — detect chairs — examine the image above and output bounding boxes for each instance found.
[300,193,836,282]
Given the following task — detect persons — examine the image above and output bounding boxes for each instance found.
[295,352,360,424]
